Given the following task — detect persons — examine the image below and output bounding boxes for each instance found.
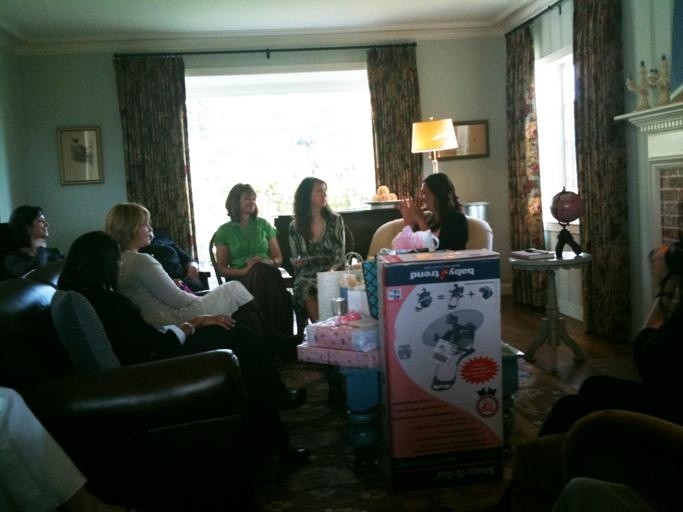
[140,231,205,295]
[214,182,294,364]
[4,207,50,277]
[397,174,466,250]
[106,203,302,344]
[287,178,345,323]
[51,232,307,468]
[497,238,682,512]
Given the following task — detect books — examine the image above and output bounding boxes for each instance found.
[512,249,552,260]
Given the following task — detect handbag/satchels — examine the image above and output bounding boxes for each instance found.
[315,251,372,322]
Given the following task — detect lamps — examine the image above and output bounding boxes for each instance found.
[409,116,459,175]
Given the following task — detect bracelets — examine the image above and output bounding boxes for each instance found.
[328,269,334,273]
[418,224,428,231]
[271,259,279,265]
[654,292,673,300]
[182,322,195,336]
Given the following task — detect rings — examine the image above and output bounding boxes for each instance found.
[220,316,226,322]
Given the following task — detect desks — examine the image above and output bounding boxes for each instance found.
[507,252,594,374]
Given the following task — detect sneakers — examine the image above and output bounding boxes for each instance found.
[277,386,307,412]
[272,435,308,465]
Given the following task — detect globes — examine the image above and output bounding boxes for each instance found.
[551,187,585,257]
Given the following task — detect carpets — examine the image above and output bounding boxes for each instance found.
[252,353,580,510]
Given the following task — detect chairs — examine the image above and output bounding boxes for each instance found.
[242,199,498,341]
[510,407,683,511]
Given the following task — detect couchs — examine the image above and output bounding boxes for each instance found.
[0,221,252,511]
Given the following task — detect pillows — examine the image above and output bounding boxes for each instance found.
[550,478,655,511]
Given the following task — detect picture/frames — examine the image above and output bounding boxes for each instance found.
[436,120,491,161]
[55,126,103,185]
